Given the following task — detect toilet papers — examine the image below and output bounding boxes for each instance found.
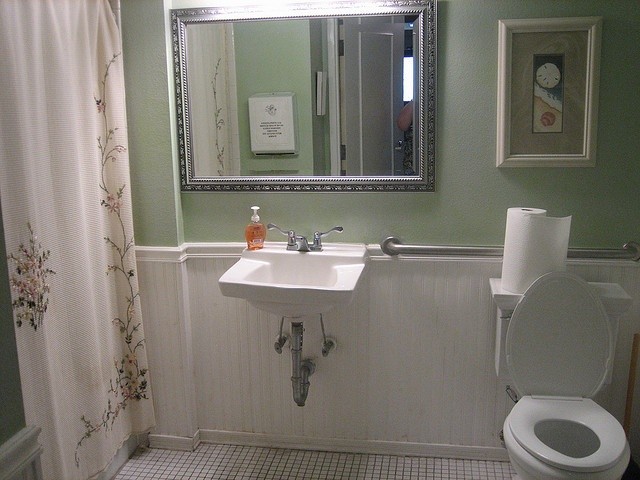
[500,205,572,295]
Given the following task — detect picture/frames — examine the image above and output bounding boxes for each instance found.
[496,16,603,169]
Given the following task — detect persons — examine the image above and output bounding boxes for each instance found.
[398,100,414,173]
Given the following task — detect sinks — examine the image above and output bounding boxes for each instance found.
[220,243,369,321]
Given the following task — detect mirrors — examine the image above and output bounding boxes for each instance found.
[167,0,440,196]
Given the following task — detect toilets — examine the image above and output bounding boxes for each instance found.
[487,277,631,480]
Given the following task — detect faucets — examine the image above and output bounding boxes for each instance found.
[292,233,312,252]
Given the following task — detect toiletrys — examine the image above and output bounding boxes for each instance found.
[245,205,267,251]
